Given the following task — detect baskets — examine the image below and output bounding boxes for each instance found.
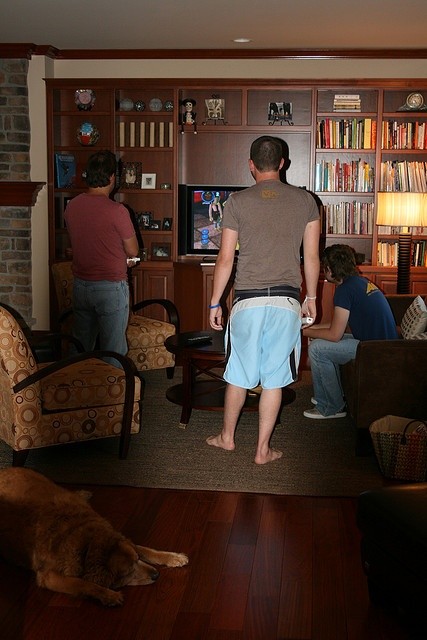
[368,415,427,481]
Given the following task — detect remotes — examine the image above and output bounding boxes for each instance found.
[187,335,212,344]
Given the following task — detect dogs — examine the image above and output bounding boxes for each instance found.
[0,466,191,608]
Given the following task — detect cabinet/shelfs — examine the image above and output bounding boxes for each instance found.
[41,75,427,363]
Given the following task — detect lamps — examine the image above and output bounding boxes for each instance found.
[375,191,427,293]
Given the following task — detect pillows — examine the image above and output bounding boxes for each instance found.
[398,295,427,343]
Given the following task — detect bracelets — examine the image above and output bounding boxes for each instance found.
[304,294,319,303]
[208,303,220,308]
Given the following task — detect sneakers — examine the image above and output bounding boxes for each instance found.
[310,393,348,405]
[303,404,348,419]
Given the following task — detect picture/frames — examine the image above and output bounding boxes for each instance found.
[140,173,156,191]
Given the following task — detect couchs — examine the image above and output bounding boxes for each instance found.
[48,257,179,380]
[340,295,426,459]
[0,304,140,470]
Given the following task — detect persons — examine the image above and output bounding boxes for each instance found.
[178,98,198,135]
[298,244,399,420]
[64,149,139,370]
[208,197,223,228]
[205,135,320,465]
[164,220,169,226]
[124,169,136,184]
[156,247,168,256]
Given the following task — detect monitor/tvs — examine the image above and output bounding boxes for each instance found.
[178,183,252,256]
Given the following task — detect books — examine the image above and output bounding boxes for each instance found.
[381,120,427,150]
[380,159,427,193]
[319,200,373,235]
[332,94,362,113]
[377,226,427,236]
[377,240,427,267]
[315,118,376,149]
[315,158,374,193]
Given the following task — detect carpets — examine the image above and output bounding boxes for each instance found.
[0,362,427,497]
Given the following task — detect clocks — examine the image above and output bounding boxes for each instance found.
[406,92,424,111]
